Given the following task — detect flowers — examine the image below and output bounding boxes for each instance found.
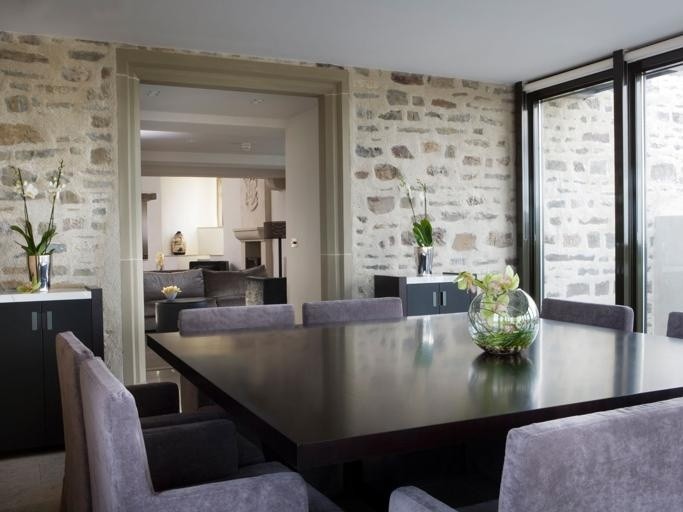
[8,160,65,255]
[452,265,533,352]
[398,176,431,246]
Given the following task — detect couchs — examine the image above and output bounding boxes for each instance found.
[144,265,270,332]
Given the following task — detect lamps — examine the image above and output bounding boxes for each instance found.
[265,221,286,278]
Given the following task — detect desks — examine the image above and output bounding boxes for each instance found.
[155,297,218,332]
[144,308,683,510]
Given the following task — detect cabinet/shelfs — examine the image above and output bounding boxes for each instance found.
[374,272,476,316]
[0,285,104,461]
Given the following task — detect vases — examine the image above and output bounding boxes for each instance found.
[467,289,539,353]
[415,246,432,276]
[27,255,51,291]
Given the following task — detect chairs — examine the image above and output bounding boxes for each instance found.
[57,330,229,507]
[541,298,634,333]
[178,303,296,333]
[302,298,403,325]
[666,311,682,340]
[81,358,310,511]
[385,395,681,511]
